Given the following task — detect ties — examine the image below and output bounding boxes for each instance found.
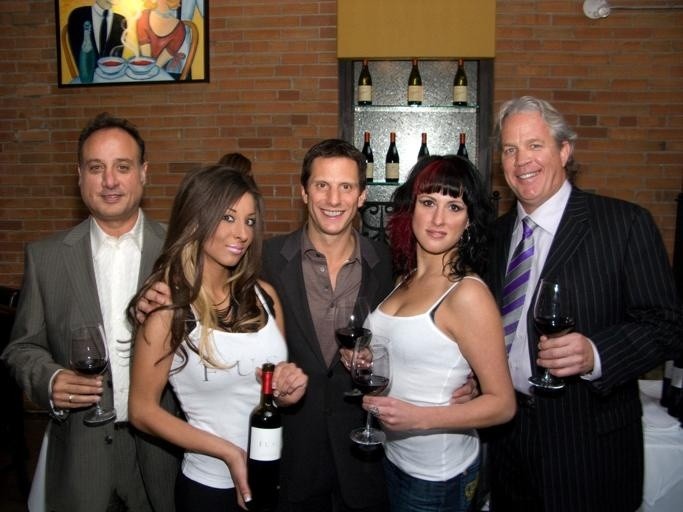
[99,10,109,55]
[500,218,538,359]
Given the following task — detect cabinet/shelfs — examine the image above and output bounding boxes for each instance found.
[339,57,486,244]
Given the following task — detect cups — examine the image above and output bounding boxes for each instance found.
[127,57,157,75]
[97,56,127,76]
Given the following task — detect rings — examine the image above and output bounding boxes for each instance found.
[68,394,73,403]
[368,407,378,417]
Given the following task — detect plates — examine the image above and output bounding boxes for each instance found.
[95,66,126,81]
[126,66,160,79]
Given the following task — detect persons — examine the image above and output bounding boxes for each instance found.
[216,152,254,180]
[100,139,480,512]
[0,112,187,512]
[126,166,308,512]
[66,0,128,80]
[337,154,516,512]
[135,0,187,75]
[468,95,679,512]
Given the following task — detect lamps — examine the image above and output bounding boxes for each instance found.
[584,0,683,21]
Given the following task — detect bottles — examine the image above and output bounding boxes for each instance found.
[454,57,468,108]
[386,132,399,183]
[457,132,470,159]
[357,58,374,106]
[362,131,374,185]
[246,363,286,494]
[407,60,423,104]
[418,132,430,158]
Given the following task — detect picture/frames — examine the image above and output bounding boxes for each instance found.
[55,0,209,89]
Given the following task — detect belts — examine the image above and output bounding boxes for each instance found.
[513,390,538,410]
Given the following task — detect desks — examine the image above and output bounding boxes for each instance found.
[637,377,683,511]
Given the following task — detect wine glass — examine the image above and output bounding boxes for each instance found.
[348,348,394,448]
[334,296,374,397]
[528,277,578,390]
[67,324,118,425]
